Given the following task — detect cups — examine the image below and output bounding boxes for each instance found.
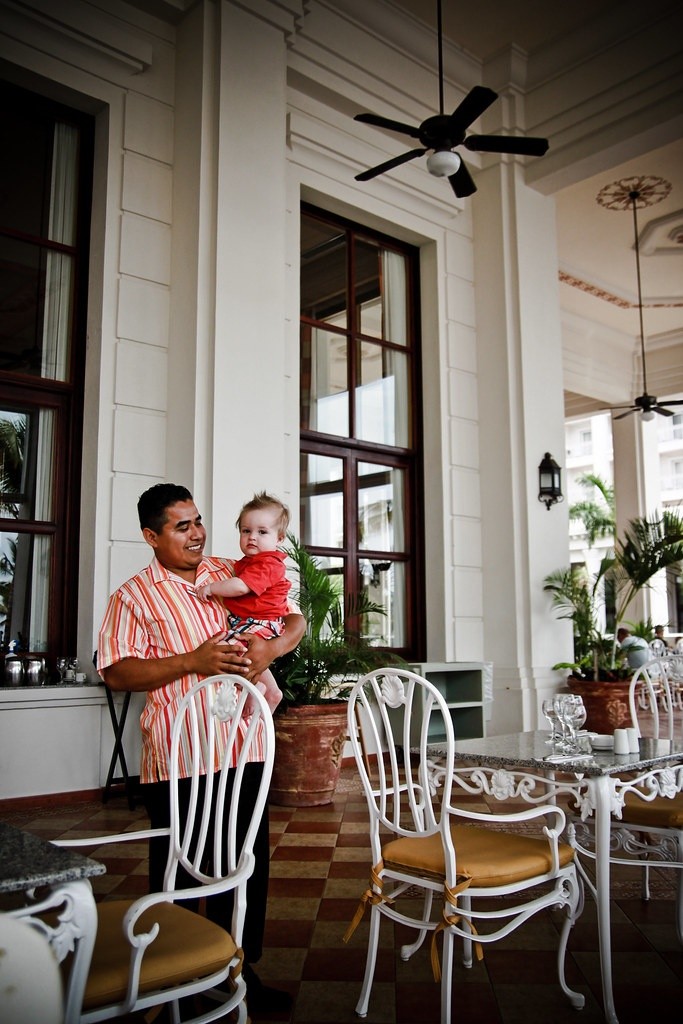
[625,728,639,753]
[614,729,629,754]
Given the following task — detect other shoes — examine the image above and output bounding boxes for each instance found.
[215,963,294,1016]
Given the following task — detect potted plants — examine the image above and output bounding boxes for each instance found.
[542,510,683,734]
[268,529,416,809]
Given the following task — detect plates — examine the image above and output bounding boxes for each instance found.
[592,746,614,750]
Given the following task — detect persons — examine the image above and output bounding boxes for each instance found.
[617,624,675,679]
[196,492,291,720]
[96,482,293,1024]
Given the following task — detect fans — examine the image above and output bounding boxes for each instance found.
[598,190,683,421]
[353,0,549,198]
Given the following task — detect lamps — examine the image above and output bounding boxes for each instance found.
[538,452,565,511]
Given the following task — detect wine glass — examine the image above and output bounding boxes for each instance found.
[564,706,586,751]
[553,694,574,746]
[542,700,560,744]
[561,696,583,748]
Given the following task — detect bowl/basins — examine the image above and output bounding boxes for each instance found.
[589,735,613,746]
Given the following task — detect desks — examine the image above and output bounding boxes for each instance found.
[409,730,683,1024]
[0,823,107,1024]
[384,661,494,750]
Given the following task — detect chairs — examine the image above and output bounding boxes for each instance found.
[632,638,683,714]
[347,667,584,1024]
[565,656,683,902]
[32,674,275,1024]
